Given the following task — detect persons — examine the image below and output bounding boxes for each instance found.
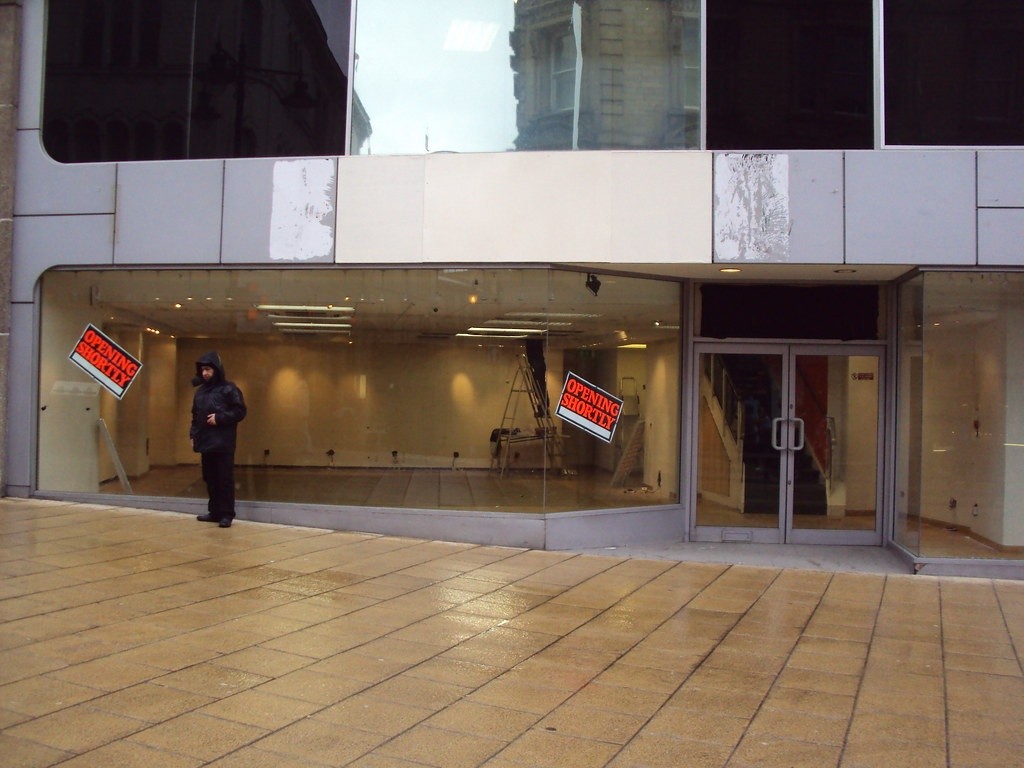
[191,351,246,527]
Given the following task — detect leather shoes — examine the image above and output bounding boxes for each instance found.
[196,512,232,528]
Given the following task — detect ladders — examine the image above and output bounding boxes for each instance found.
[490,347,574,481]
